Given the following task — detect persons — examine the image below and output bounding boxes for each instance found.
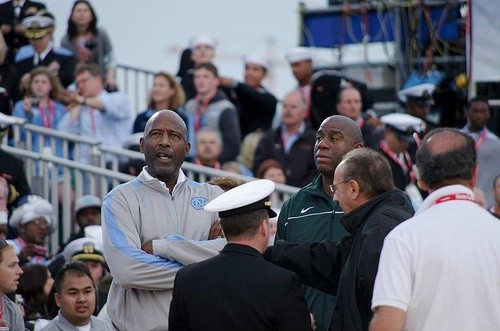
[168,179,315,330]
[369,127,500,330]
[263,147,416,331]
[100,110,229,331]
[0,0,500,331]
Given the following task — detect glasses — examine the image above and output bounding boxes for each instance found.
[0,238,9,250]
[31,217,48,231]
[328,180,349,193]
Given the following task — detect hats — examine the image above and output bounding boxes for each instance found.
[63,238,105,263]
[74,195,101,219]
[83,226,103,243]
[191,37,216,50]
[7,194,53,232]
[398,83,436,106]
[23,16,54,38]
[284,47,314,63]
[243,54,269,72]
[381,112,424,142]
[204,179,277,218]
